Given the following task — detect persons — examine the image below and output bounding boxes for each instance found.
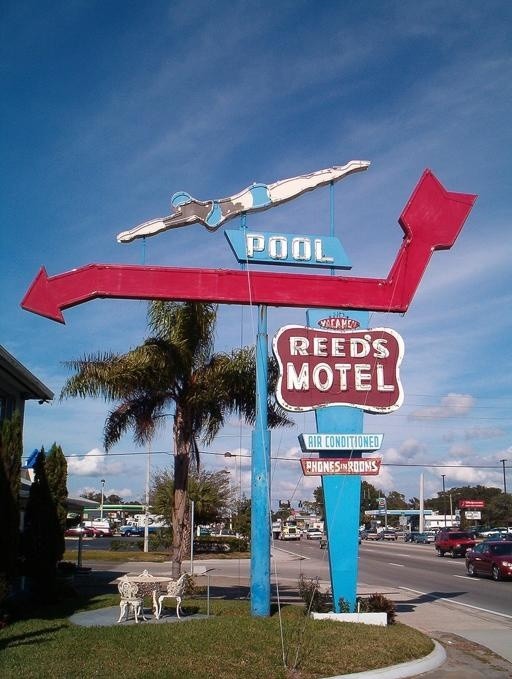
[116,160,371,244]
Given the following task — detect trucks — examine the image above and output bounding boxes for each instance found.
[119,514,170,537]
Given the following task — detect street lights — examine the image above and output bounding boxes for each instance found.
[100,479,105,519]
[441,474,446,527]
[500,459,507,494]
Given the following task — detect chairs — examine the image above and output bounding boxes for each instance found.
[117,569,191,623]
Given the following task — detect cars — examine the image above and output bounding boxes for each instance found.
[359,527,511,581]
[272,522,327,549]
[64,514,115,537]
[197,527,236,537]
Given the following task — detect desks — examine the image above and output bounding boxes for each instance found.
[109,576,173,616]
